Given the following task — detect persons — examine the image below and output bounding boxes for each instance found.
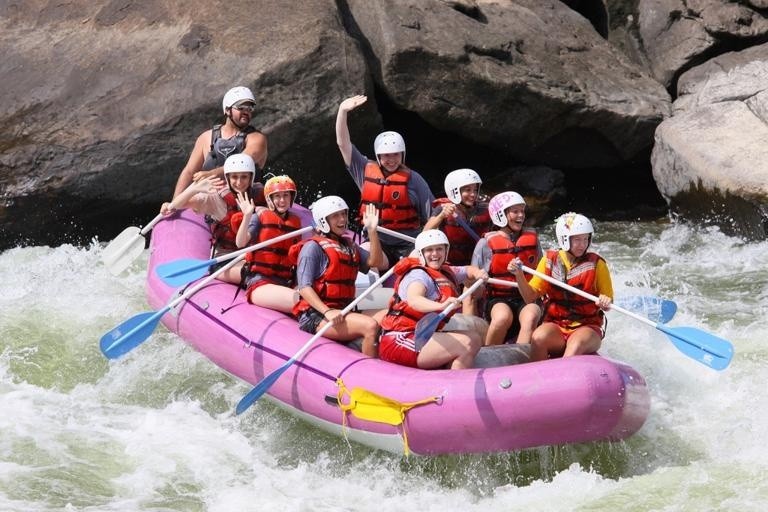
[422,169,500,294]
[160,87,268,218]
[234,176,304,319]
[336,93,436,289]
[469,190,546,347]
[506,212,612,364]
[377,229,489,373]
[289,196,389,360]
[175,153,267,287]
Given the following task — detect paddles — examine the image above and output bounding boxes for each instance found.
[100,179,200,276]
[155,226,315,287]
[234,267,396,416]
[518,263,733,372]
[100,250,247,361]
[414,278,484,355]
[488,277,677,325]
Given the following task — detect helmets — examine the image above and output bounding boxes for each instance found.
[374,132,406,167]
[488,191,526,226]
[224,153,256,187]
[415,230,450,266]
[264,175,297,211]
[556,213,593,251]
[223,86,256,116]
[312,196,349,233]
[444,169,482,204]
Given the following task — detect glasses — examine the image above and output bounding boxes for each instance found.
[238,105,253,112]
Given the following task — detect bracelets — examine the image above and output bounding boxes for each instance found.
[323,307,334,315]
